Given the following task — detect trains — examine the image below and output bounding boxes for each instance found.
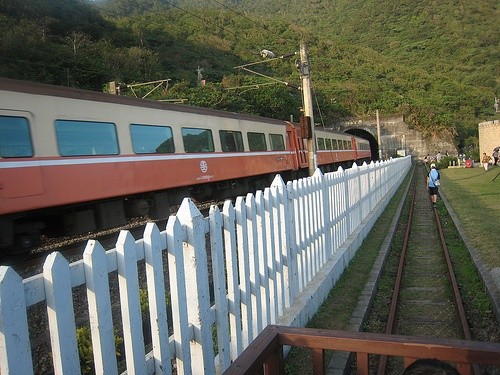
[0,76,372,264]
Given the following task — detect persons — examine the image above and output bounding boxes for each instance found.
[437,151,441,162]
[493,149,498,165]
[483,153,490,171]
[424,154,430,164]
[465,159,473,167]
[445,151,449,157]
[427,164,440,207]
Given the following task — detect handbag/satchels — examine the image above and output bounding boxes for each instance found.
[433,180,441,187]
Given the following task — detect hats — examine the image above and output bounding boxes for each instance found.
[431,163,435,168]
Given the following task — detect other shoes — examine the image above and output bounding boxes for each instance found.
[431,202,437,208]
